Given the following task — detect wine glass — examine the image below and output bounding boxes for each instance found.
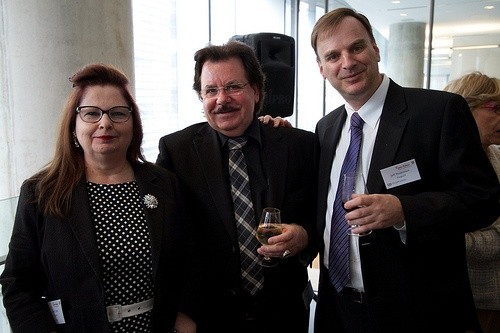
[255,207,283,267]
[341,171,373,236]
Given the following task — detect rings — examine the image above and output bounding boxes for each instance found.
[283,250,292,258]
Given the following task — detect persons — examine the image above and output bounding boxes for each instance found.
[0,62,201,333]
[442,71,500,333]
[258,8,500,333]
[155,40,320,333]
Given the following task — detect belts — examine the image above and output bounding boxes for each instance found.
[342,287,368,305]
[106,298,153,322]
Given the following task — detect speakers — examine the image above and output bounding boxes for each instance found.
[230,33,295,119]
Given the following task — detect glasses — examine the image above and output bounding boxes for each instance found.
[76,105,135,124]
[477,102,500,114]
[199,81,250,96]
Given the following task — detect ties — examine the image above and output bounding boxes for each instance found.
[227,136,267,300]
[328,112,365,293]
